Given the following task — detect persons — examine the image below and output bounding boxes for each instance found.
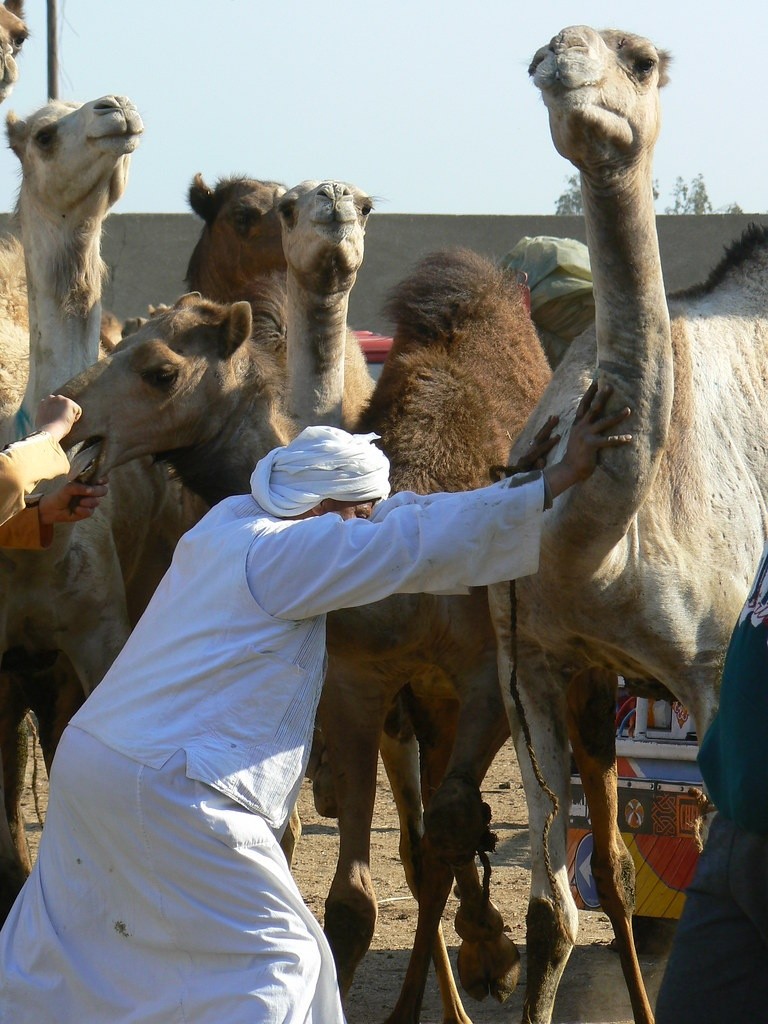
[653,548,768,1024]
[0,374,636,1024]
[0,393,111,551]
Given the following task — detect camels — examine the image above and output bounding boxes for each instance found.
[0,0,143,928]
[59,177,558,1024]
[486,25,767,1022]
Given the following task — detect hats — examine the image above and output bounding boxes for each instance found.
[249,425,391,518]
